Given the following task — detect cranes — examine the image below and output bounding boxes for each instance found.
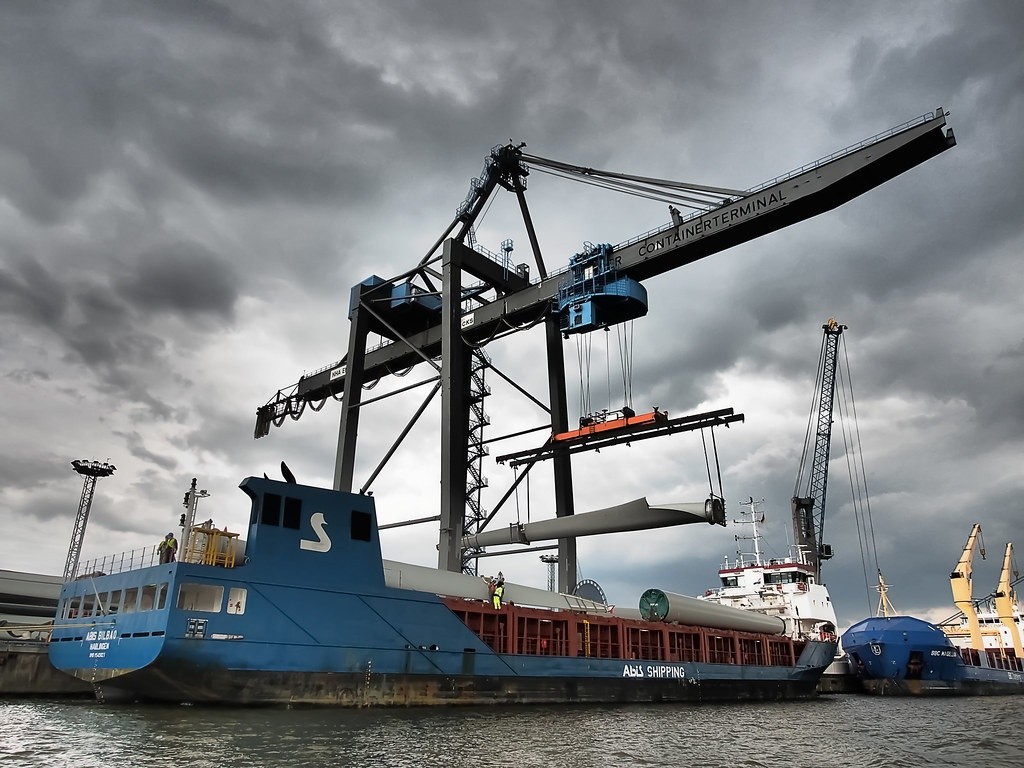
[254,96,958,566]
[991,542,1024,658]
[949,523,988,650]
[791,322,851,583]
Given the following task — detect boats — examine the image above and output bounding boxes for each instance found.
[685,494,855,695]
[43,459,848,709]
[841,523,1024,697]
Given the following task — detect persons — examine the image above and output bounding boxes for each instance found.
[157,533,178,565]
[482,577,505,610]
